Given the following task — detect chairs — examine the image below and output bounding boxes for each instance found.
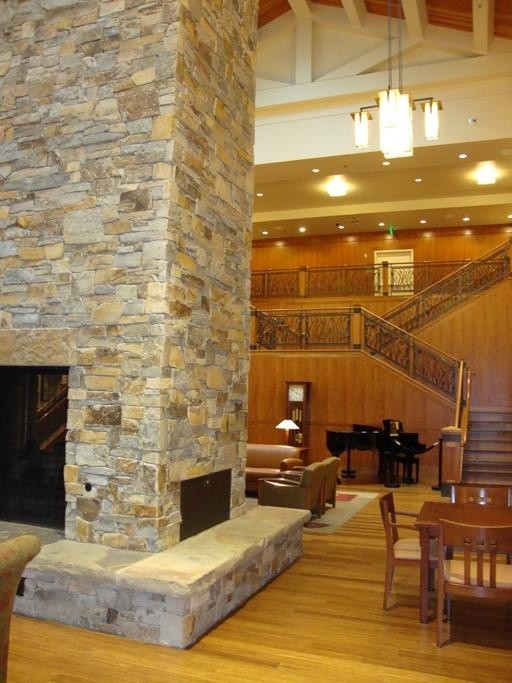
[378,484,512,647]
[0,534,41,683]
[258,457,341,518]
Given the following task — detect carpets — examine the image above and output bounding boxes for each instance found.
[243,489,379,534]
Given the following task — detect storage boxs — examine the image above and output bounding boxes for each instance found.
[274,419,300,430]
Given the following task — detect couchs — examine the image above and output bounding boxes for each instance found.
[246,443,307,492]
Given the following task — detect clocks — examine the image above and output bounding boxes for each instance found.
[284,380,312,448]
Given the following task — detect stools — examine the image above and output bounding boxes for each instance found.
[392,457,419,482]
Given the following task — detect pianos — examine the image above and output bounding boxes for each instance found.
[324,419,425,488]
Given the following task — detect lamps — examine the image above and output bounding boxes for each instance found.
[349,0,443,160]
